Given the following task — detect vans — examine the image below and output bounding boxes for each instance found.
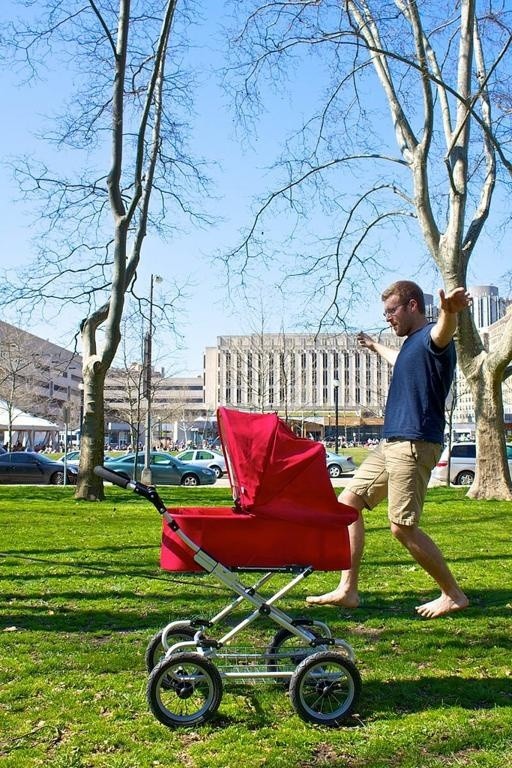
[433,443,512,485]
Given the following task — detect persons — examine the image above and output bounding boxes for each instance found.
[304,281,473,620]
[3,433,475,452]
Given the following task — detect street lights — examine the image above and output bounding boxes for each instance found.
[332,378,344,455]
[141,274,163,485]
[77,380,85,454]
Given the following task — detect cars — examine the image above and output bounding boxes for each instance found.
[324,448,356,478]
[59,449,116,476]
[104,452,216,486]
[155,449,228,478]
[1,450,78,484]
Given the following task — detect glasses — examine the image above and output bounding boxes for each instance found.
[383,303,408,316]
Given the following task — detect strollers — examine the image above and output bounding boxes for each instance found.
[91,407,359,728]
